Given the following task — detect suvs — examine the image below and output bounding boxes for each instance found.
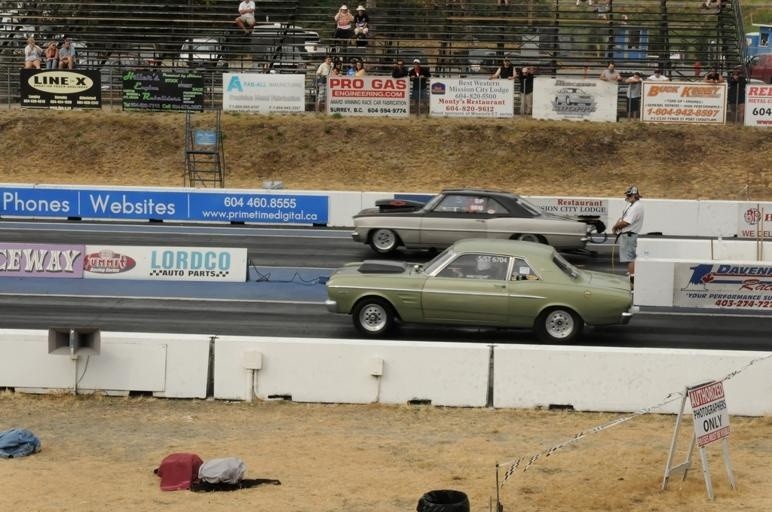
[339,46,370,71]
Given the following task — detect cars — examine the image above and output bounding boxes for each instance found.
[555,88,596,107]
[67,38,228,95]
[460,50,521,73]
[378,50,431,77]
[746,53,772,85]
[263,45,307,74]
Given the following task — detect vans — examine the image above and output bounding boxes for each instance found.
[252,25,321,50]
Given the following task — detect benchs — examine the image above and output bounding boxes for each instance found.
[0,0,751,106]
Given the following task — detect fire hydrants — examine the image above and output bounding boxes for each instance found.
[694,62,702,77]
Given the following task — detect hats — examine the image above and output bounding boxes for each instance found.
[413,59,420,63]
[625,186,639,195]
[504,56,511,61]
[340,5,365,11]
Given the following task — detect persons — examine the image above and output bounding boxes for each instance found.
[43,40,59,70]
[353,5,369,47]
[518,68,534,115]
[234,0,257,36]
[624,72,646,121]
[314,56,334,111]
[613,184,644,292]
[645,69,669,81]
[58,39,76,70]
[704,65,725,83]
[391,60,407,78]
[346,57,362,77]
[693,57,702,76]
[728,69,747,127]
[408,58,431,117]
[331,59,343,77]
[487,56,517,80]
[703,0,722,9]
[600,62,622,82]
[333,4,355,46]
[354,61,368,77]
[23,38,41,70]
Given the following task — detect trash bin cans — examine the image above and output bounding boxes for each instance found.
[418,490,470,512]
